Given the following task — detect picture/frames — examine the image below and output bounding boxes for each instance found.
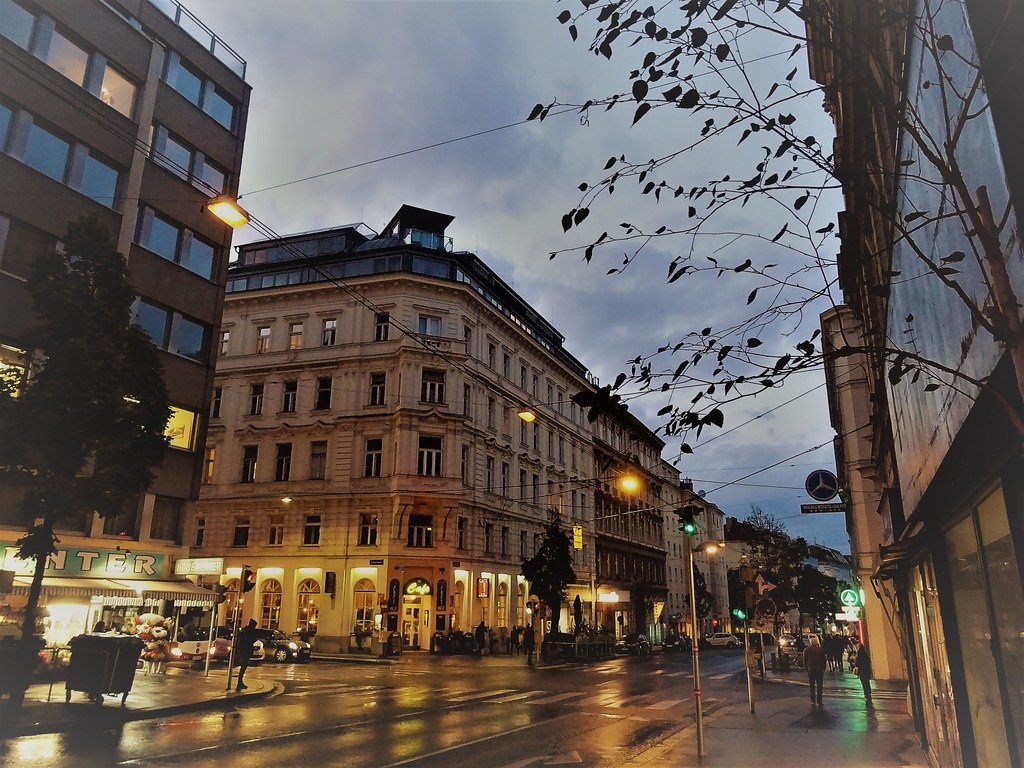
[477,578,489,598]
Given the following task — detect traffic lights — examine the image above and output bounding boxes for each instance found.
[672,507,693,534]
[242,570,256,594]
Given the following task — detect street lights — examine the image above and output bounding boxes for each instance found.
[689,540,723,759]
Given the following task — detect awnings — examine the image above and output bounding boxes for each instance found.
[7,576,221,607]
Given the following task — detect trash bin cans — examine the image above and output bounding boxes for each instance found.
[63,633,145,708]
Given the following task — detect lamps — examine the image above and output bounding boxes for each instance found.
[280,488,293,503]
[116,546,132,554]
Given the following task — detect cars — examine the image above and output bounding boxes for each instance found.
[662,635,694,652]
[192,624,265,663]
[614,633,650,656]
[776,634,821,660]
[254,627,312,662]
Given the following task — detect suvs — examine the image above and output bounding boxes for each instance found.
[702,633,737,649]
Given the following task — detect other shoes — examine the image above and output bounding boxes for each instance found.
[810,702,815,705]
[237,681,248,689]
[818,703,825,707]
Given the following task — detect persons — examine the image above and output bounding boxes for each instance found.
[472,621,692,665]
[754,633,872,706]
[235,618,257,689]
[91,618,133,632]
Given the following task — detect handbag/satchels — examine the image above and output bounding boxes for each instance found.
[853,666,859,675]
[753,654,761,659]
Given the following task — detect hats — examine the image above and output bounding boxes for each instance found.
[249,618,257,628]
[809,638,818,646]
